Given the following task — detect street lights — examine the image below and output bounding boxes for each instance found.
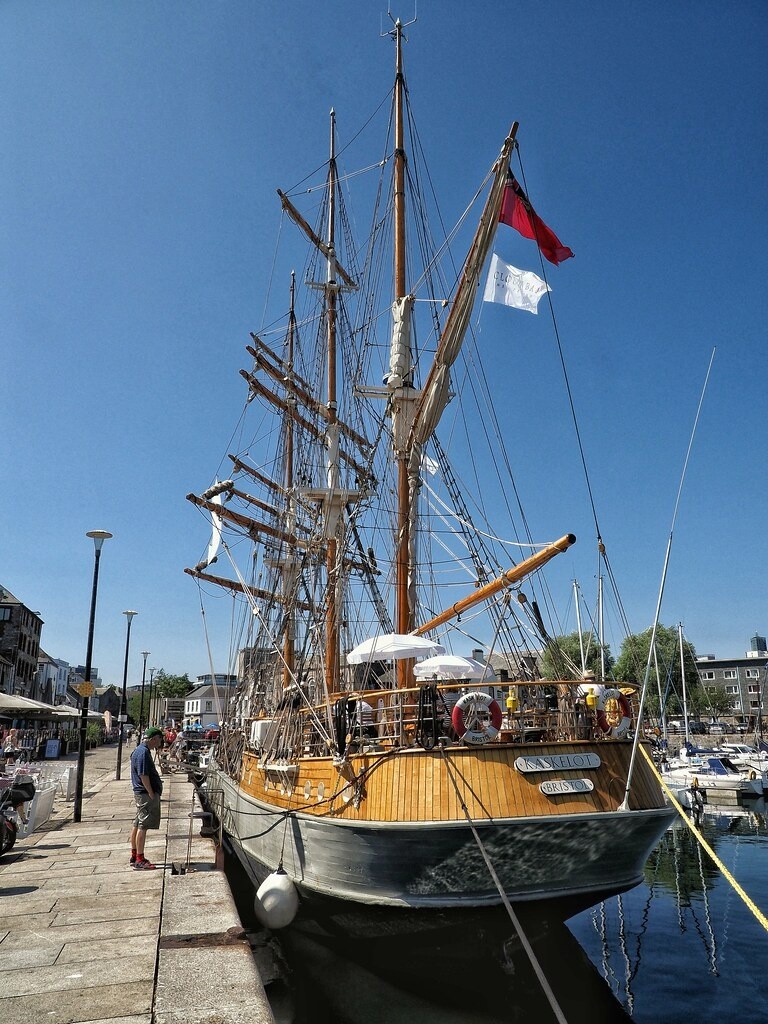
[147,668,159,730]
[71,528,112,822]
[115,611,137,780]
[115,610,139,780]
[138,651,153,746]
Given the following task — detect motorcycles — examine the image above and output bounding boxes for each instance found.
[0,774,36,858]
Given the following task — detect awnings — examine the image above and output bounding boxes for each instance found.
[182,719,189,722]
[191,717,198,722]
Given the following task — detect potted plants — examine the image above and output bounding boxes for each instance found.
[86,721,102,750]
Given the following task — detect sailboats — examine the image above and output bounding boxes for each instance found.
[191,12,693,948]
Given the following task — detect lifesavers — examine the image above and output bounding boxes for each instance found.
[452,691,503,745]
[596,689,632,739]
[749,770,757,781]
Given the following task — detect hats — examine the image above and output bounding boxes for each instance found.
[581,670,600,679]
[143,727,166,739]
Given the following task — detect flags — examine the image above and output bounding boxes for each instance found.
[498,165,575,267]
[483,253,552,314]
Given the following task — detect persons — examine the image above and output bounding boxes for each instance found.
[438,679,465,739]
[130,728,163,872]
[333,693,378,738]
[3,728,20,764]
[126,725,177,751]
[576,670,607,712]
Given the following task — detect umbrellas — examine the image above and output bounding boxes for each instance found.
[183,722,219,733]
[413,655,493,679]
[0,693,117,729]
[346,631,446,746]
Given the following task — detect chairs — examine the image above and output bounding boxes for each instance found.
[362,711,376,723]
[448,700,471,742]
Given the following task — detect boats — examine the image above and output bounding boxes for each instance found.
[642,622,768,818]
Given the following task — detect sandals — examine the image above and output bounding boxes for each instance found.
[133,859,156,870]
[130,857,135,865]
